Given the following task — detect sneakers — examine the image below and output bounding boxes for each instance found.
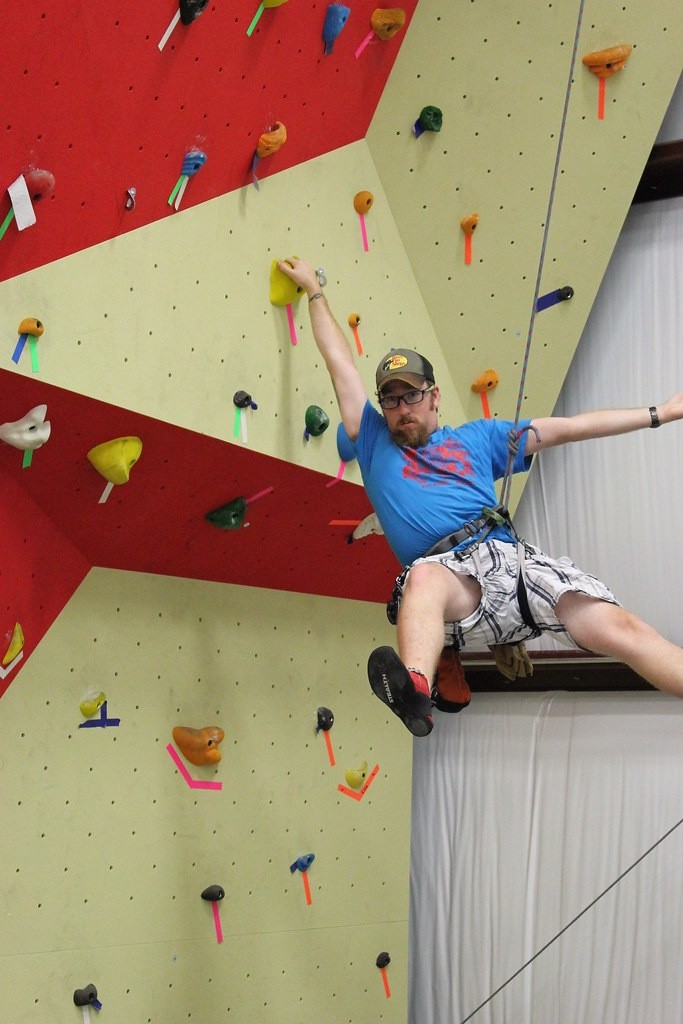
[368,649,432,737]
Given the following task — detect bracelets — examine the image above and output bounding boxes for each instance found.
[649,407,660,428]
[308,293,323,302]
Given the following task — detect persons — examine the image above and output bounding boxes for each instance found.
[278,257,683,736]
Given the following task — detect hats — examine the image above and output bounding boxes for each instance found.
[375,348,435,397]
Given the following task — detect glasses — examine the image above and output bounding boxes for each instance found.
[377,384,435,410]
